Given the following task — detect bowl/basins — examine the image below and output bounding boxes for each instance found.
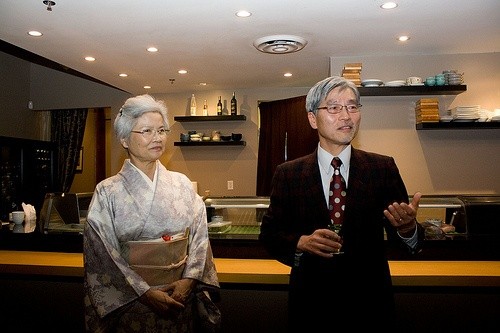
[231,133,242,141]
[220,135,232,141]
[212,138,221,142]
[427,219,442,227]
[361,79,383,87]
[211,216,223,221]
[445,72,462,86]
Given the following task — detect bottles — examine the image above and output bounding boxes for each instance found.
[188,130,196,136]
[217,95,222,116]
[203,100,208,116]
[190,94,196,116]
[231,91,237,115]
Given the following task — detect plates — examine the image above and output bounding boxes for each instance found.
[410,84,425,86]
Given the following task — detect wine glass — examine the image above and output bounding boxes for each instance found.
[327,224,344,255]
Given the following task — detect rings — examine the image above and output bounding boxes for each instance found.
[398,219,403,221]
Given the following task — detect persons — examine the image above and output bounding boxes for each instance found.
[259,76,425,333]
[83,94,221,333]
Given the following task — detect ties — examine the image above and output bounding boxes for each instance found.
[329,156,346,245]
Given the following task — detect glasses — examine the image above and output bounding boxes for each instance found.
[131,128,171,139]
[309,103,362,114]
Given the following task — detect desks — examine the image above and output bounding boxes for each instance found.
[0,250,500,333]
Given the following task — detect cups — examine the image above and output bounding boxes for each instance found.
[181,133,189,142]
[408,77,422,84]
[12,211,25,225]
[435,74,445,86]
[427,77,435,86]
[13,225,24,233]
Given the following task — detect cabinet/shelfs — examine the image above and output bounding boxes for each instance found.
[174,115,247,146]
[357,85,500,130]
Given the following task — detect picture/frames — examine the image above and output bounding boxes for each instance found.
[75,147,83,173]
